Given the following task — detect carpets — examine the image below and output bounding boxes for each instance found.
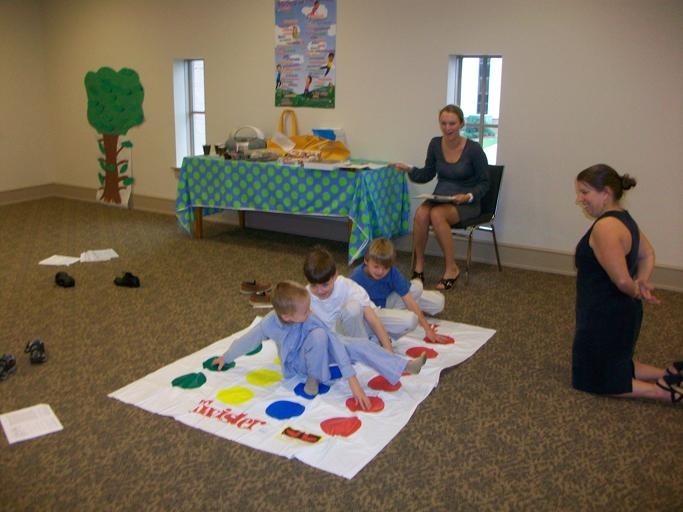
[106,302,496,479]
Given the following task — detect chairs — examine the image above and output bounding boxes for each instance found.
[413,164,505,285]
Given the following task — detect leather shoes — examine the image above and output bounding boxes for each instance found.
[115,273,140,288]
[55,271,75,288]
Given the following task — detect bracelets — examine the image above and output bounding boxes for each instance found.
[466,192,474,204]
[408,164,413,173]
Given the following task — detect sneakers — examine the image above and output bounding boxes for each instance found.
[25,340,47,363]
[0,353,16,381]
[240,279,273,294]
[249,292,273,307]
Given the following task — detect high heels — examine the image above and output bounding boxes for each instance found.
[657,359,683,407]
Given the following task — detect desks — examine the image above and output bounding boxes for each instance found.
[183,155,411,267]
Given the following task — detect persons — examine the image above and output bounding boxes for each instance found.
[320,53,334,76]
[307,1,320,22]
[303,246,419,353]
[275,63,283,89]
[394,102,492,290]
[303,74,313,96]
[570,162,683,404]
[350,237,449,344]
[211,278,428,410]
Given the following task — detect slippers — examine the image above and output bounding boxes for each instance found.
[436,276,461,291]
[410,270,424,285]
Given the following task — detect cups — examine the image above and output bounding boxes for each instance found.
[202,144,211,155]
[214,143,227,157]
